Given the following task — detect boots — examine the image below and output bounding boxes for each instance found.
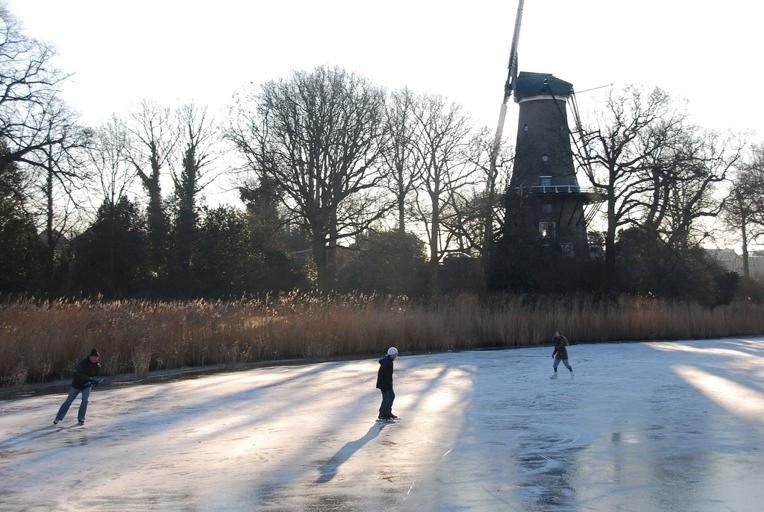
[550,372,558,379]
[379,410,397,419]
[570,370,575,376]
[78,418,84,423]
[53,416,59,424]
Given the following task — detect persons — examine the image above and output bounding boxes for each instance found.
[53,348,102,424]
[550,330,573,379]
[376,347,398,419]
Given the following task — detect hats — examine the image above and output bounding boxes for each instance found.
[90,349,99,356]
[385,348,399,357]
